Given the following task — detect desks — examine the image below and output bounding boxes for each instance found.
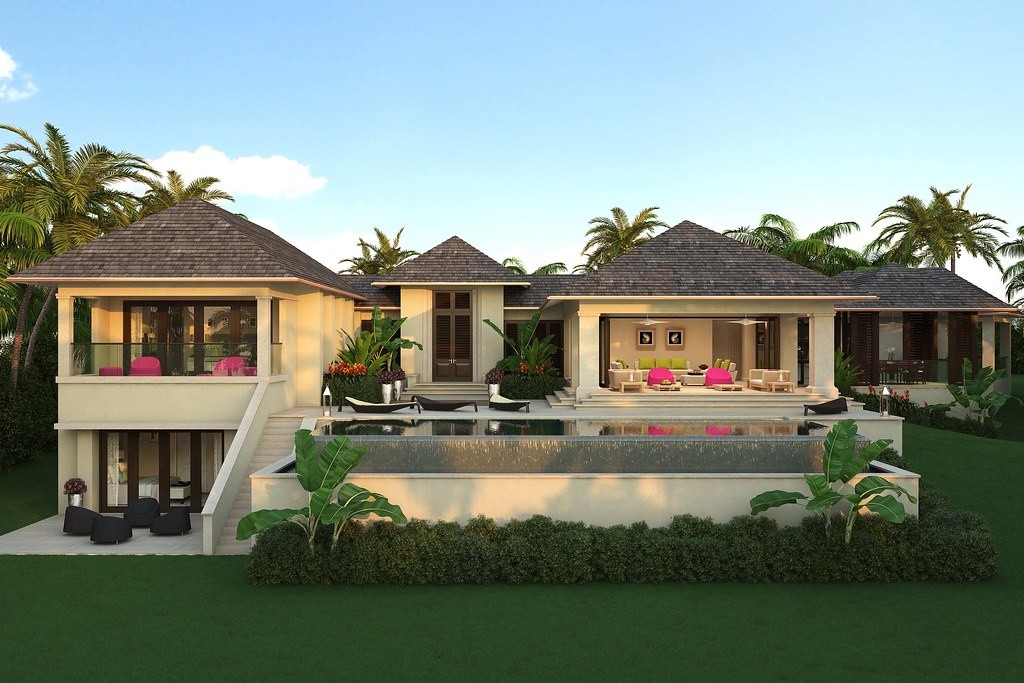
[619,381,643,394]
[681,375,705,386]
[768,382,795,393]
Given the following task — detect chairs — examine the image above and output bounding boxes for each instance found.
[213,356,245,376]
[704,367,732,388]
[648,367,674,387]
[803,398,847,416]
[130,357,162,376]
[150,506,191,535]
[123,498,162,528]
[64,506,102,537]
[90,515,132,544]
[410,396,478,412]
[490,393,530,413]
[338,397,421,414]
[879,361,927,385]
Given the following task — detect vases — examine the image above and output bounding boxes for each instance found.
[68,494,83,507]
[381,380,403,403]
[489,384,501,397]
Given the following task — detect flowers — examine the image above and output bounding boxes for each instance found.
[380,369,406,384]
[484,369,504,384]
[887,346,896,359]
[63,478,87,494]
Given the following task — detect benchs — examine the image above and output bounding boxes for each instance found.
[170,481,191,504]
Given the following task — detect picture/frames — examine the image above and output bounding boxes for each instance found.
[636,327,656,351]
[664,327,686,351]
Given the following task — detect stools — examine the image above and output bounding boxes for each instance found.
[99,367,122,376]
[244,367,257,376]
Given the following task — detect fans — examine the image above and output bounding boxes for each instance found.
[727,315,767,326]
[632,318,669,327]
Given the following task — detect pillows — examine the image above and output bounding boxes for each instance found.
[616,358,622,363]
[655,358,670,369]
[621,360,627,369]
[722,360,730,370]
[640,358,655,368]
[672,358,687,369]
[714,359,722,368]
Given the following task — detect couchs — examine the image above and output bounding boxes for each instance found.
[702,362,738,383]
[748,368,791,392]
[635,360,694,382]
[608,358,643,388]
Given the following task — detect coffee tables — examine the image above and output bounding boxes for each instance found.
[652,384,682,391]
[714,382,742,391]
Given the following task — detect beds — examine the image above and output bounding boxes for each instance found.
[118,458,180,505]
